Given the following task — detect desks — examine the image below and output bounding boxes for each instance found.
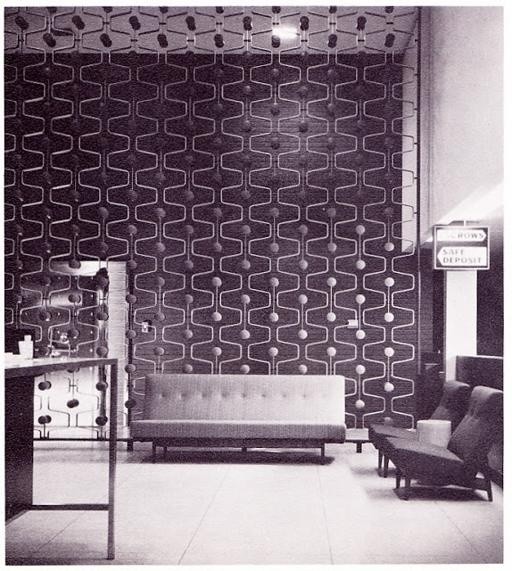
[5,356,120,561]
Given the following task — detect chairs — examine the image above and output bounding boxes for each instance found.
[381,385,503,502]
[368,379,471,478]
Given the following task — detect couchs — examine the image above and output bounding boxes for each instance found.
[129,373,347,466]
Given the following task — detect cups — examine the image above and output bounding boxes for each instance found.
[17,340,34,361]
